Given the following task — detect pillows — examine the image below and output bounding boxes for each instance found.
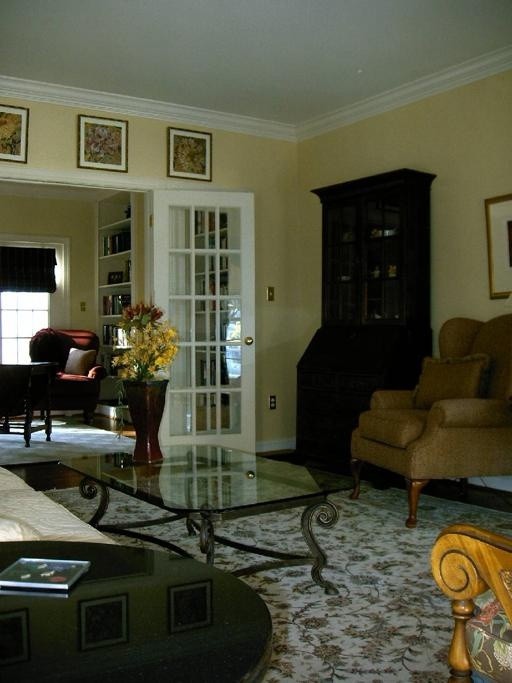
[64,347,96,375]
[410,352,491,409]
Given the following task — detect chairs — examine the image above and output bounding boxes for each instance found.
[349,314,512,528]
[29,327,109,424]
[430,523,512,682]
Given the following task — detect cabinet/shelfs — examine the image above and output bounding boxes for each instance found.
[94,190,230,408]
[295,168,437,463]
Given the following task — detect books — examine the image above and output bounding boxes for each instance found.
[195,210,230,408]
[103,231,131,375]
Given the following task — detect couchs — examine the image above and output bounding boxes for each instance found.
[0,464,123,547]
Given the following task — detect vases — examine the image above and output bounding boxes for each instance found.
[120,379,169,463]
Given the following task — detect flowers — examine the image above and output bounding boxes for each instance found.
[111,301,180,439]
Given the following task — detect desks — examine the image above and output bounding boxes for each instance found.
[0,361,52,448]
[0,541,273,682]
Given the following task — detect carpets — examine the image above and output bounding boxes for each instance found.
[43,464,511,682]
[0,417,136,465]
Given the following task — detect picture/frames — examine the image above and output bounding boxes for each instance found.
[484,194,512,299]
[77,113,129,172]
[0,103,29,164]
[166,126,212,182]
